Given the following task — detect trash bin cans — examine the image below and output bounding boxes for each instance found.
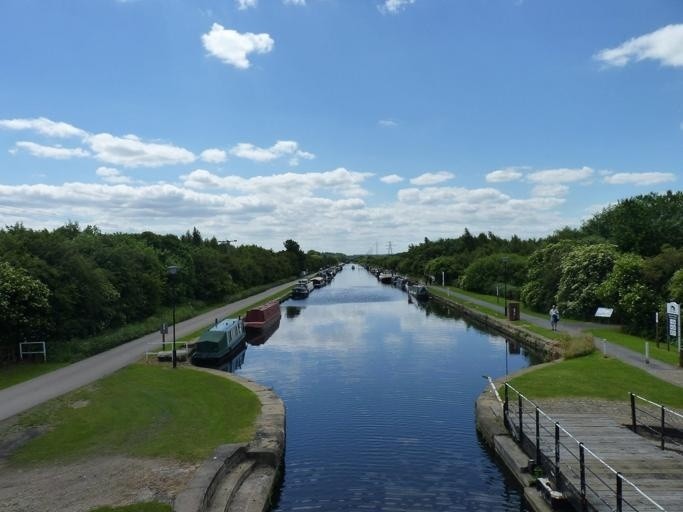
[509,303,520,321]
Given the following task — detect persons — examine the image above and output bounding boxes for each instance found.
[548,305,561,330]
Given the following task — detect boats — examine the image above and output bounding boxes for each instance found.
[352,265,355,269]
[291,265,342,299]
[191,301,281,363]
[368,266,430,300]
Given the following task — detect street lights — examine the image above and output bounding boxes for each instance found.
[503,257,512,316]
[167,266,177,368]
